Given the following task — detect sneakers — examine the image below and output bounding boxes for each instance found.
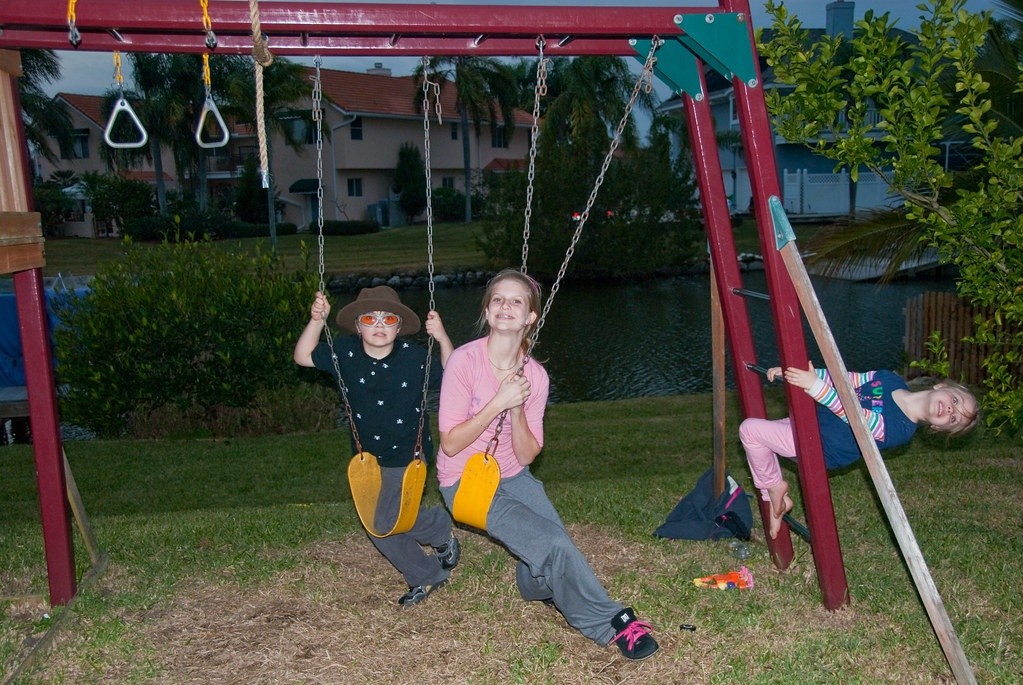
[399,576,448,607]
[609,608,659,661]
[540,598,557,608]
[432,528,460,571]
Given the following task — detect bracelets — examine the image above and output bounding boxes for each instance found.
[473,415,487,430]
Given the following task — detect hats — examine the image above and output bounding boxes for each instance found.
[336,285,421,337]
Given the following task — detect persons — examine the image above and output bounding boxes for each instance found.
[293,286,460,603]
[738,361,980,539]
[434,269,659,660]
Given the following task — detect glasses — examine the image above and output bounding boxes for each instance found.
[491,272,542,298]
[357,312,401,327]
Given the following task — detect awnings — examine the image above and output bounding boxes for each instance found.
[62,181,110,199]
[289,179,321,194]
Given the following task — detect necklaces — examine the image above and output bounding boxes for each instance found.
[488,356,518,370]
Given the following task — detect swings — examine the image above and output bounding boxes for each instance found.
[450,32,665,538]
[310,53,443,541]
